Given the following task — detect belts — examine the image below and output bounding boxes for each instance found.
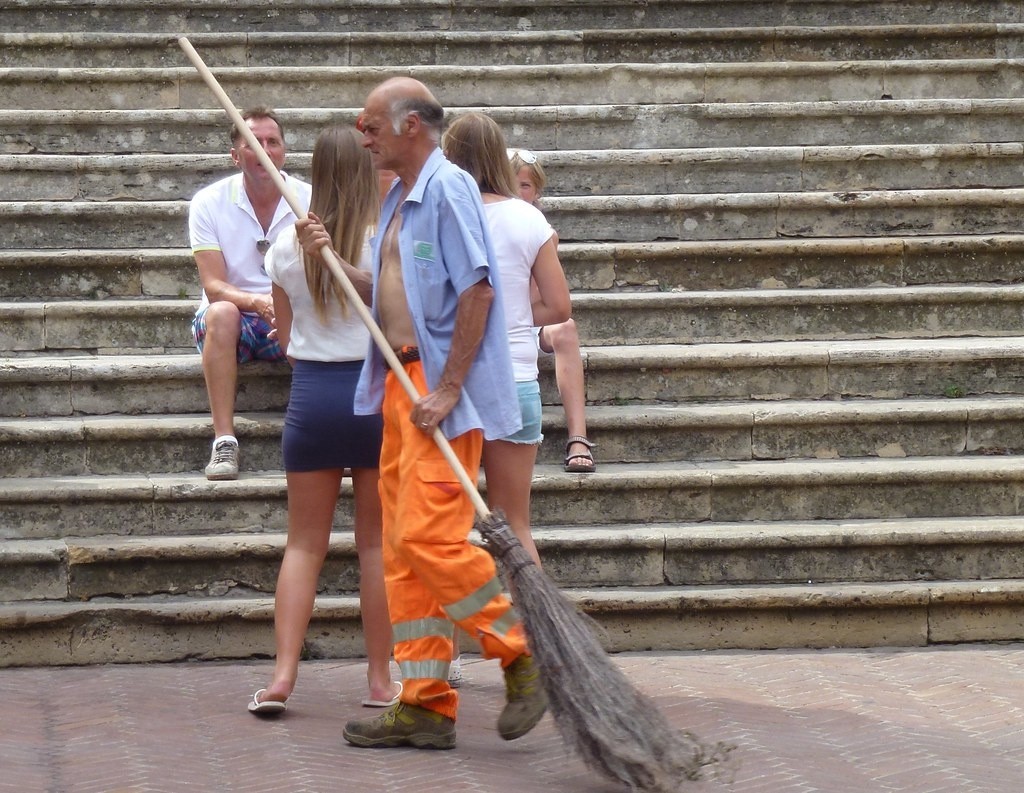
[385,348,420,368]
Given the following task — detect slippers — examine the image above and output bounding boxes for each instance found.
[248,689,289,712]
[362,680,403,707]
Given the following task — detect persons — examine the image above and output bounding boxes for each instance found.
[440,113,571,610]
[341,76,553,751]
[248,123,404,717]
[187,103,312,480]
[506,146,599,473]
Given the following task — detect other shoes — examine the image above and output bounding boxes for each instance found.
[448,666,461,687]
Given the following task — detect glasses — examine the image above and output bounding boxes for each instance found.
[257,240,272,276]
[506,148,537,164]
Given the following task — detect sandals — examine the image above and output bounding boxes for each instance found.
[564,436,599,471]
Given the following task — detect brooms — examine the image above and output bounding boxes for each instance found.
[177,36,701,793]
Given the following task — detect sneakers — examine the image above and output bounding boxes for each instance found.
[205,440,241,480]
[343,701,456,749]
[498,654,550,740]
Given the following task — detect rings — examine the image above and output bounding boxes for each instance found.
[421,422,428,426]
